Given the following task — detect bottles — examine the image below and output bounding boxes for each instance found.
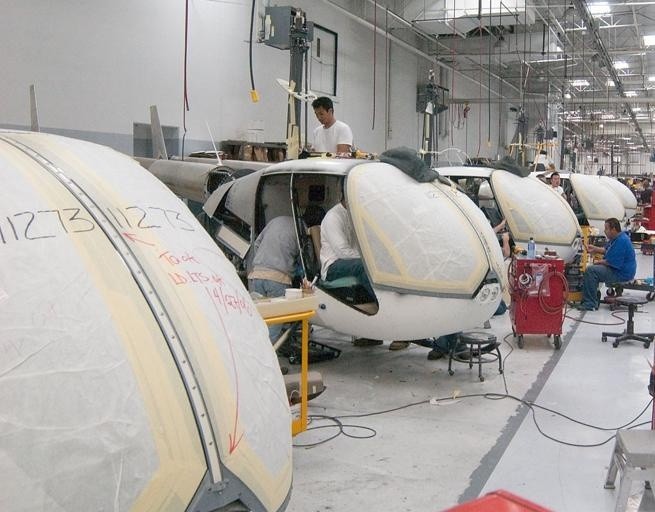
[527,237,536,259]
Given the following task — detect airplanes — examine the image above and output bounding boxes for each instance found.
[430,149,581,269]
[585,174,638,221]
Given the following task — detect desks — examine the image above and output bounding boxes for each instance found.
[265,310,318,436]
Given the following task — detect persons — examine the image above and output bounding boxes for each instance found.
[247,177,410,358]
[637,172,655,191]
[493,217,510,257]
[427,332,473,360]
[551,172,567,199]
[310,97,352,155]
[533,150,555,171]
[577,218,636,310]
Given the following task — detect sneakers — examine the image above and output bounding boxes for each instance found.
[577,304,598,311]
[354,337,472,360]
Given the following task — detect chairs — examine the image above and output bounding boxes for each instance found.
[481,204,507,233]
[309,225,362,305]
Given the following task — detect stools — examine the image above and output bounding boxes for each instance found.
[603,428,655,512]
[601,295,655,349]
[447,331,503,381]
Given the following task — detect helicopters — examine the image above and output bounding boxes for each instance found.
[133,106,510,346]
[0,130,295,512]
